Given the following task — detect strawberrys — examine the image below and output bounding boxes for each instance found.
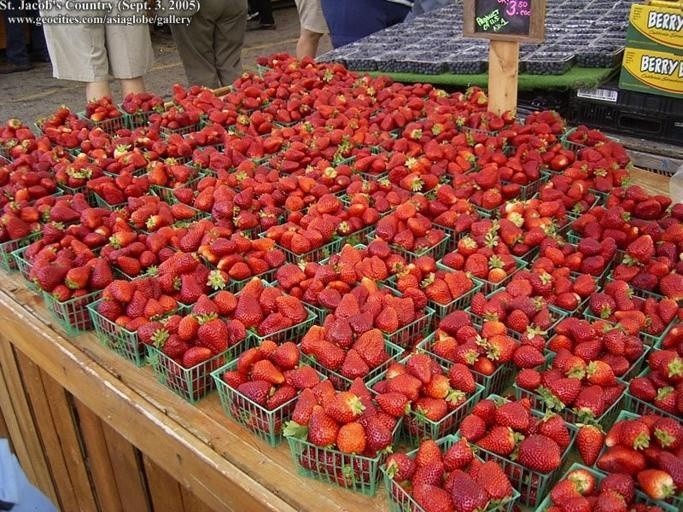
[0,55,683,512]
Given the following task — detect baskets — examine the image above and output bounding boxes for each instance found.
[282,390,405,499]
[593,409,683,512]
[44,258,683,422]
[209,346,328,449]
[454,393,580,509]
[0,62,683,252]
[534,463,680,512]
[378,433,521,512]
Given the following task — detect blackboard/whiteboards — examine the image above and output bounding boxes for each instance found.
[463,0,545,44]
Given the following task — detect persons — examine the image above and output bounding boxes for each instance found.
[0,0,50,75]
[166,1,251,90]
[36,1,156,107]
[293,0,330,63]
